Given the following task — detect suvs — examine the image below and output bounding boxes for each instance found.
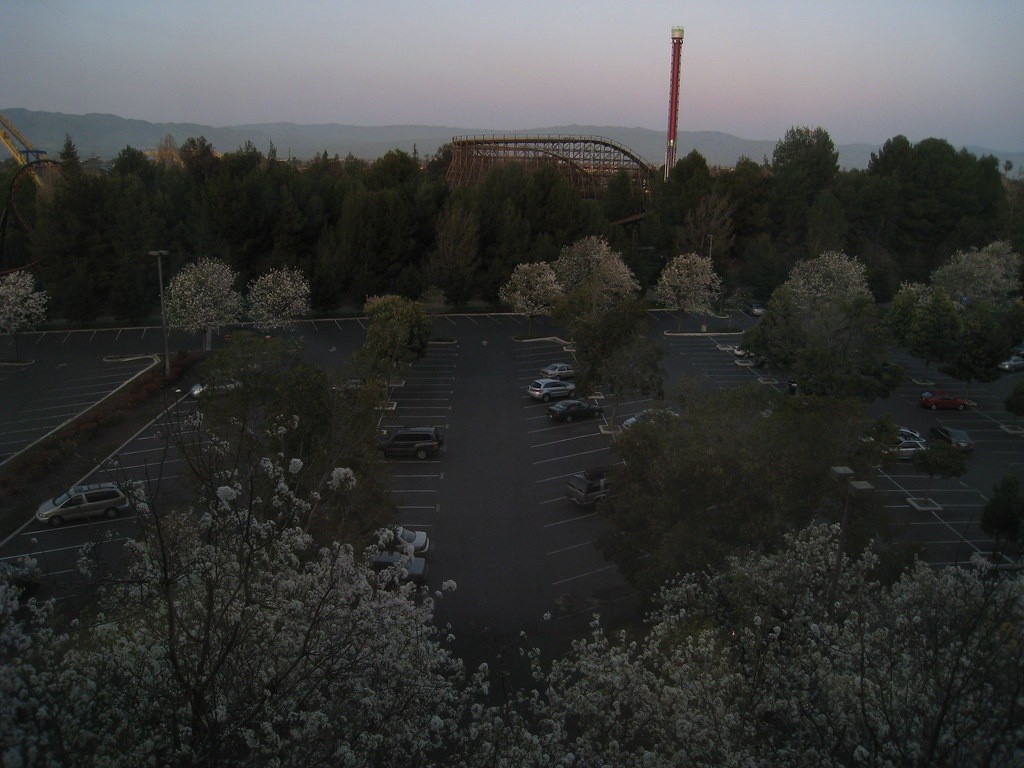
[528,379,576,401]
[384,426,441,459]
[36,483,131,528]
[566,468,616,509]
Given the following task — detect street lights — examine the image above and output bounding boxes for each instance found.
[149,250,170,378]
[825,466,875,611]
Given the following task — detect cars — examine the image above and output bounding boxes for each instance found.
[876,424,930,459]
[998,354,1024,372]
[373,550,430,583]
[541,363,576,380]
[733,345,753,356]
[383,526,430,557]
[191,379,241,397]
[919,390,969,410]
[622,409,679,430]
[752,303,765,315]
[546,400,602,423]
